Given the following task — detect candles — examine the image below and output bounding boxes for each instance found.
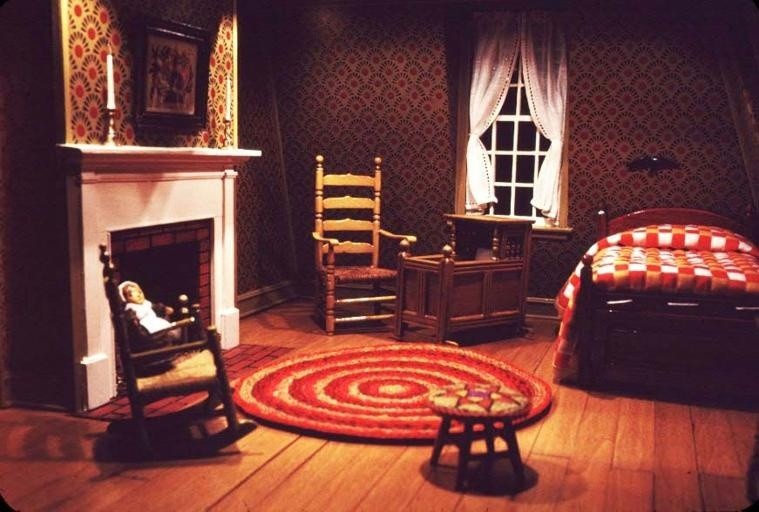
[106,54,117,109]
[225,76,231,120]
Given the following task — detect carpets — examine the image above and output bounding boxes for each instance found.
[70,343,295,423]
[233,341,554,446]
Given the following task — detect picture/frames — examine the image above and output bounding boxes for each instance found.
[132,11,211,129]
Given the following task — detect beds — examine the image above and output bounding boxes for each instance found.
[547,207,757,410]
[397,212,536,343]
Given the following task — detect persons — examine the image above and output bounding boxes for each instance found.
[118,279,173,334]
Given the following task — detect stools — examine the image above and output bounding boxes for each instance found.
[421,382,529,490]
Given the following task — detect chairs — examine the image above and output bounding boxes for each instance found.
[308,154,418,337]
[98,239,257,462]
